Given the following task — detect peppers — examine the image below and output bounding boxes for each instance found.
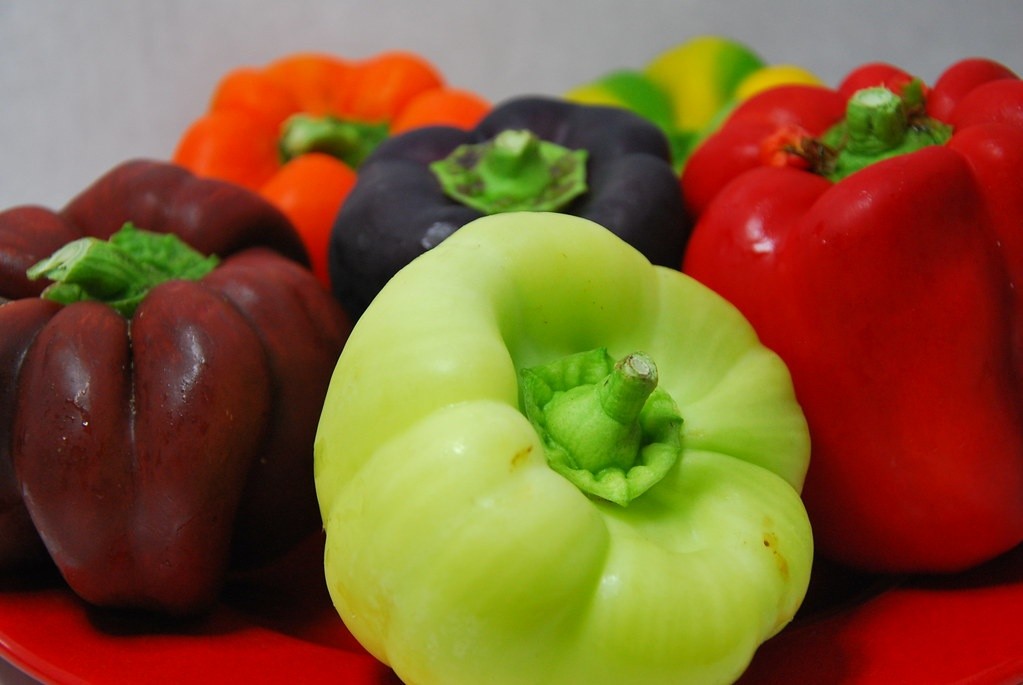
[0,38,1023,685]
